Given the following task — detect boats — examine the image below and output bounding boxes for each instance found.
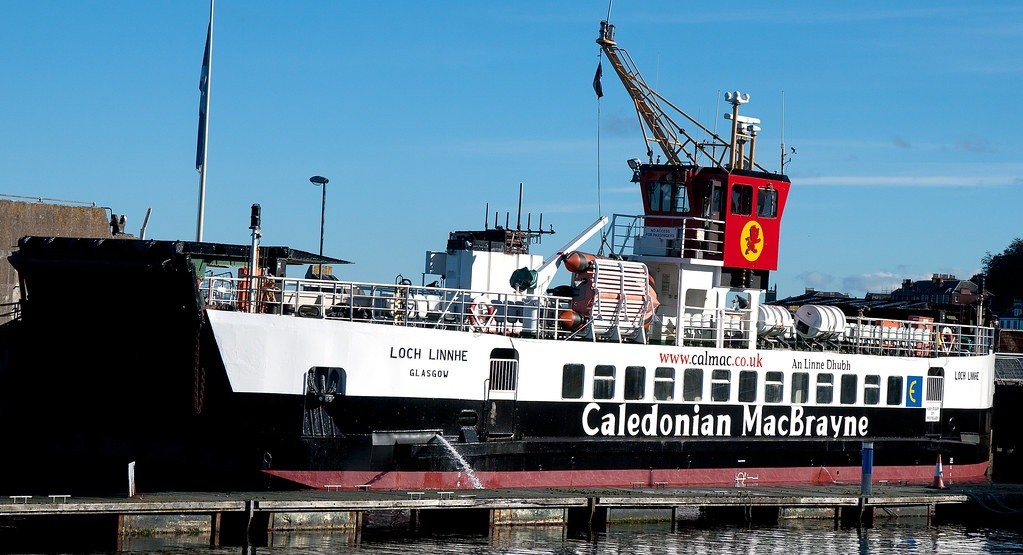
[559,251,660,337]
[1,0,995,490]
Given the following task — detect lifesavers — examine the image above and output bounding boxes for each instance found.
[469,295,494,329]
[940,326,953,351]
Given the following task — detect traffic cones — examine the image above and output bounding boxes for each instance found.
[926,454,950,490]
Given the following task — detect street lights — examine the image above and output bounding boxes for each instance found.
[309,175,329,292]
